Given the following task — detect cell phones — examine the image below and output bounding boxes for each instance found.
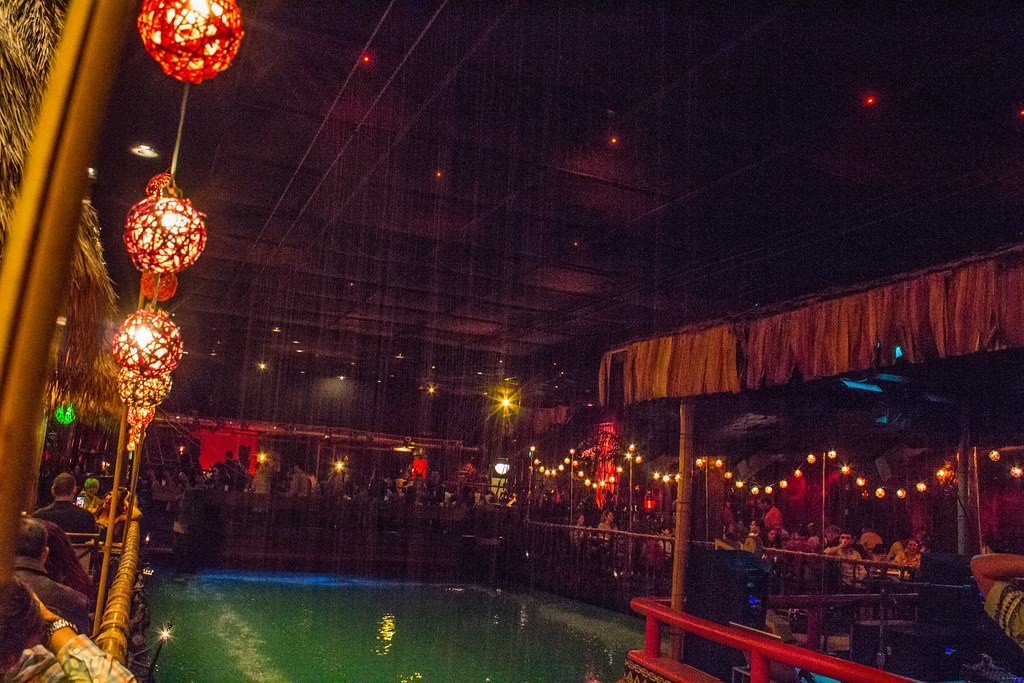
[76,496,85,508]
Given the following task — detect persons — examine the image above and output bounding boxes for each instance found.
[140,449,519,507]
[0,578,137,683]
[970,553,1024,652]
[541,485,931,623]
[13,473,142,637]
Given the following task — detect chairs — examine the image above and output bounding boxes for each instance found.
[63,530,101,588]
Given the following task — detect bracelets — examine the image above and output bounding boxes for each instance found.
[42,618,78,650]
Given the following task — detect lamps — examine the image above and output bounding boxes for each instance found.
[114,0,245,454]
